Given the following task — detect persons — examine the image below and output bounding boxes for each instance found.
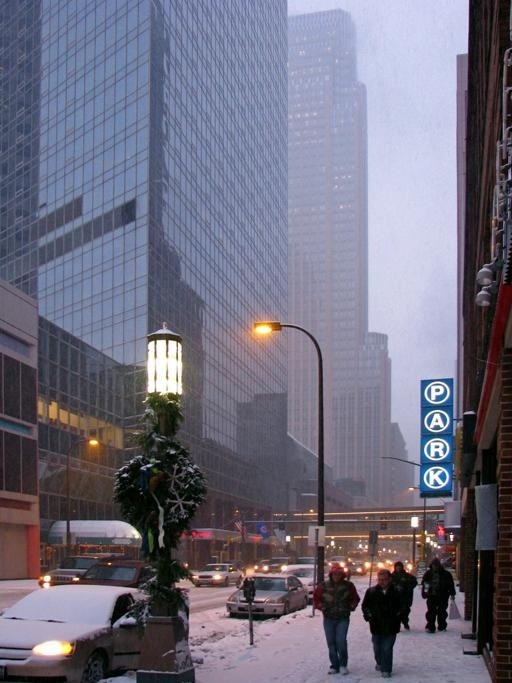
[419,558,457,631]
[313,561,361,675]
[392,560,417,631]
[358,569,408,677]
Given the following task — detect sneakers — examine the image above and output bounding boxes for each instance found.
[376,665,381,671]
[424,628,432,633]
[328,668,338,674]
[340,667,347,675]
[382,672,390,678]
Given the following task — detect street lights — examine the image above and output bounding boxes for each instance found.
[408,487,427,560]
[252,321,325,588]
[65,438,98,555]
[411,515,420,564]
[425,529,442,560]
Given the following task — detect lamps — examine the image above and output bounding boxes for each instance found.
[475,278,500,306]
[477,242,507,284]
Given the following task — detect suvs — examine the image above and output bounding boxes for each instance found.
[38,552,122,587]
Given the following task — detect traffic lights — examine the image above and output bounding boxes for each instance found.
[192,531,196,537]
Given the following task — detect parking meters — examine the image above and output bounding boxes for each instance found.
[241,576,256,644]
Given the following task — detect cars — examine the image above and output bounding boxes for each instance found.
[1,585,190,681]
[74,560,155,587]
[281,564,327,599]
[441,557,453,568]
[223,552,383,578]
[225,574,309,618]
[193,563,243,585]
